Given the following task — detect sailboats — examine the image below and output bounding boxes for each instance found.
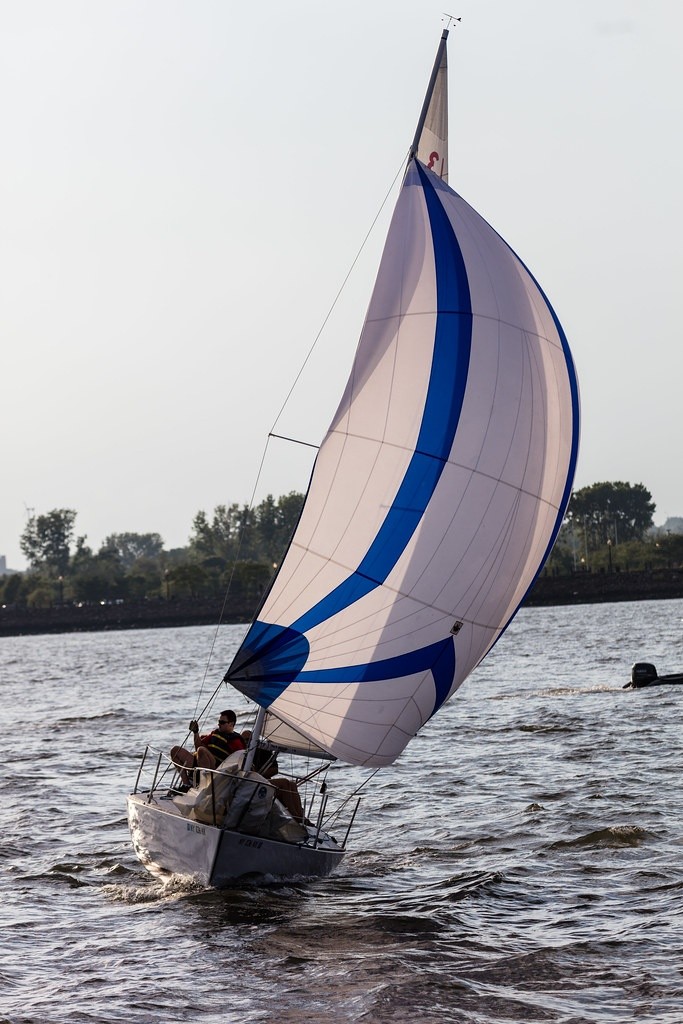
[125,8,583,893]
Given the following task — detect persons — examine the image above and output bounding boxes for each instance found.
[241,730,315,827]
[170,710,246,795]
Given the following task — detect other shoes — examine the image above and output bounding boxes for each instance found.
[172,785,194,796]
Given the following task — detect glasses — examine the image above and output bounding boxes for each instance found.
[218,720,233,725]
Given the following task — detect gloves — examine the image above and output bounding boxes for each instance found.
[189,720,199,734]
